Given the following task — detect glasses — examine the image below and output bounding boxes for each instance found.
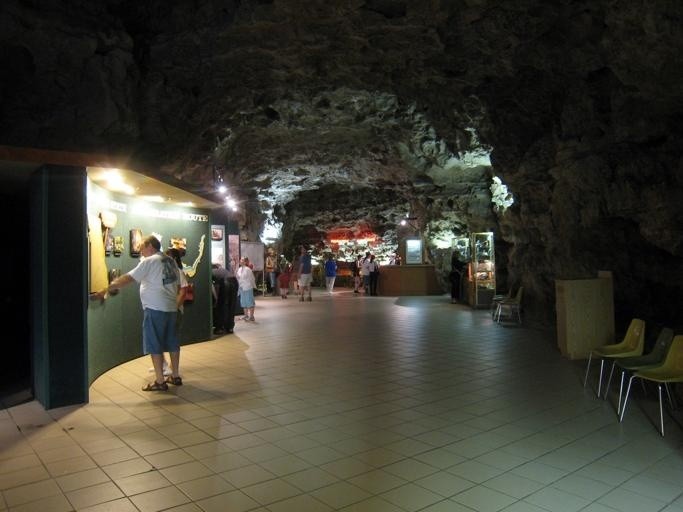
[164,376,182,386]
[142,379,168,390]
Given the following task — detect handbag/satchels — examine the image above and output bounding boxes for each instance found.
[349,261,356,271]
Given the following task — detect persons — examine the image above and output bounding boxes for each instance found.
[235,256,256,321]
[289,247,299,290]
[388,253,401,266]
[211,268,238,334]
[296,244,314,304]
[277,267,289,299]
[146,248,188,377]
[94,234,182,392]
[278,256,292,296]
[365,254,379,296]
[209,264,222,328]
[351,254,361,293]
[360,252,371,295]
[449,250,471,304]
[323,254,337,295]
[266,247,278,296]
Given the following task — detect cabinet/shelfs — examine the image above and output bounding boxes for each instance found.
[451,232,496,307]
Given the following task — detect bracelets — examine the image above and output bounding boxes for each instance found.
[103,285,111,294]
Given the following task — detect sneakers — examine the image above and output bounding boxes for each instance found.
[354,290,359,293]
[281,294,287,299]
[239,315,254,321]
[299,296,312,302]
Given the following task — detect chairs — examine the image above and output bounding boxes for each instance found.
[603,328,674,415]
[584,319,644,398]
[620,336,683,437]
[491,283,524,324]
